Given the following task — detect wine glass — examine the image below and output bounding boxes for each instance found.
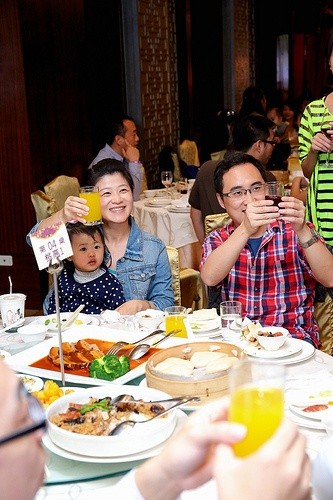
[321,106,333,168]
[161,171,173,199]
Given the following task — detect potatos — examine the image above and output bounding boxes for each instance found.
[31,380,73,407]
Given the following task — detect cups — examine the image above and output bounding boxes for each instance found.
[0,293,26,328]
[79,185,102,226]
[226,359,288,458]
[219,301,242,328]
[264,182,285,220]
[165,306,189,336]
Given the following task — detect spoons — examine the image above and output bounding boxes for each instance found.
[107,397,199,436]
[128,329,183,360]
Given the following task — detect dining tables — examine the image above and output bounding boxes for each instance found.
[0,316,333,500]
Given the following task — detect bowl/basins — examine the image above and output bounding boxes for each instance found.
[188,179,196,184]
[143,189,155,198]
[254,326,289,351]
[16,324,48,343]
[135,309,164,329]
[45,384,178,458]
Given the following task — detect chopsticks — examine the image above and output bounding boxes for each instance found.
[60,304,85,330]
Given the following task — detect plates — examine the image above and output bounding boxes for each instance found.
[144,199,191,213]
[158,314,221,338]
[40,407,189,464]
[285,406,326,430]
[28,311,101,337]
[241,337,316,365]
[4,323,211,385]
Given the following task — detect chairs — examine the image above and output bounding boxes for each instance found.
[178,139,200,167]
[31,175,204,311]
[205,213,333,357]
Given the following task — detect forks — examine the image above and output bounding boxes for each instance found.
[108,394,191,404]
[105,329,164,356]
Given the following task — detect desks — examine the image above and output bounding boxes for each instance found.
[132,194,204,270]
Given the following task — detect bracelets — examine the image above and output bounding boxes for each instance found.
[144,299,152,309]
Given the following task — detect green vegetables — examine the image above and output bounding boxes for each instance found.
[80,399,113,414]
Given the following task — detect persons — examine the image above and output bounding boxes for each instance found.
[187,80,313,309]
[298,48,333,308]
[48,221,126,315]
[88,115,147,203]
[198,154,333,352]
[42,159,176,316]
[0,355,312,500]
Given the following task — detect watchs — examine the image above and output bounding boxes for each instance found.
[298,227,320,248]
[129,160,139,164]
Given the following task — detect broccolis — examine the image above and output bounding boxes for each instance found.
[89,354,130,382]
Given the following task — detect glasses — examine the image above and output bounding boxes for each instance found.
[0,393,49,445]
[258,140,278,147]
[220,184,266,200]
